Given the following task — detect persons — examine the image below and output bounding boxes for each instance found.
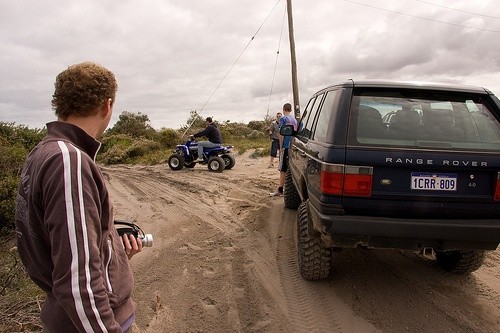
[189,117,222,162]
[14,61,142,333]
[268,113,282,167]
[269,103,299,197]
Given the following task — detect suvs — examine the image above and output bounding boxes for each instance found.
[278,78,500,282]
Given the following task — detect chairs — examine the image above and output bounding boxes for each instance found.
[426,108,454,133]
[389,102,420,136]
[356,104,385,138]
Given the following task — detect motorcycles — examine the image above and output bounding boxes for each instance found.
[168,136,235,173]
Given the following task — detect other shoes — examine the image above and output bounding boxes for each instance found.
[269,191,283,197]
[193,158,204,162]
[268,164,274,167]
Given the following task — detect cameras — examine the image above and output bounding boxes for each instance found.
[116,228,153,251]
